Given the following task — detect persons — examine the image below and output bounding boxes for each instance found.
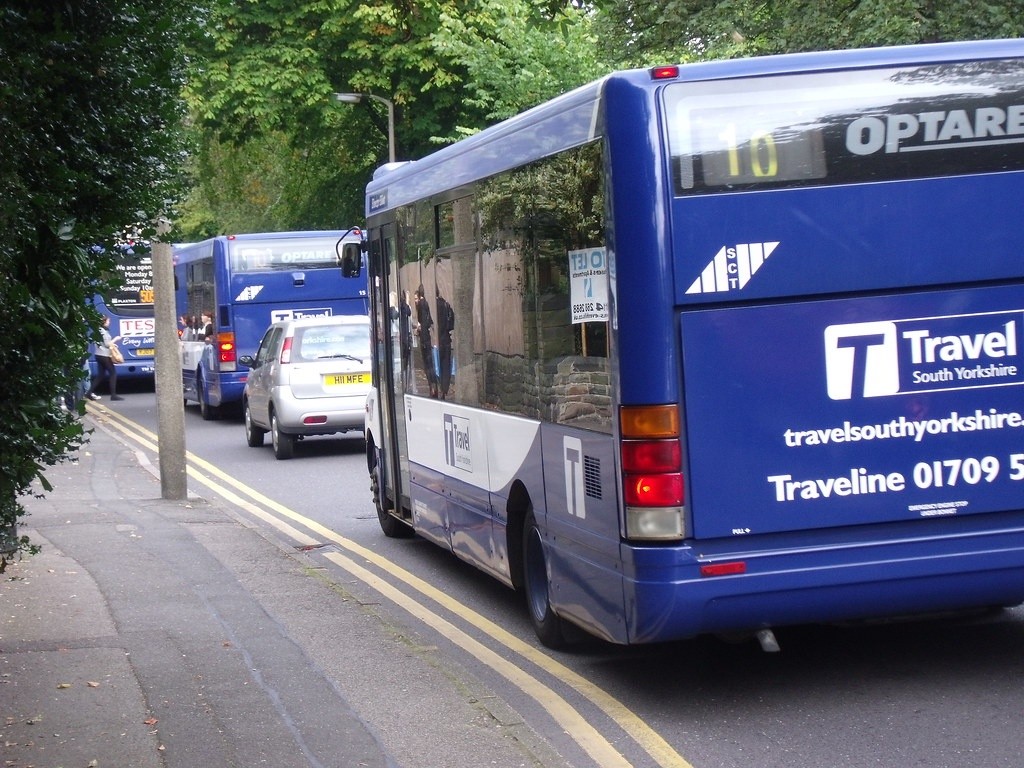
[389,285,454,400]
[179,310,214,342]
[55,315,124,420]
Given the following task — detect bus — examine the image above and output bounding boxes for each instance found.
[335,36,1024,652]
[172,230,370,420]
[80,237,155,394]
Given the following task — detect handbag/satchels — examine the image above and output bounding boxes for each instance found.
[449,348,456,380]
[109,342,124,363]
[431,345,440,376]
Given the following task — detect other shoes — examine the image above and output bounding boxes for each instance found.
[90,394,101,401]
[86,393,95,401]
[110,397,124,401]
[429,389,436,397]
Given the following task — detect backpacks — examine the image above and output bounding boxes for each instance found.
[443,302,454,332]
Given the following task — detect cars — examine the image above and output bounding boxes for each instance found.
[238,315,373,459]
